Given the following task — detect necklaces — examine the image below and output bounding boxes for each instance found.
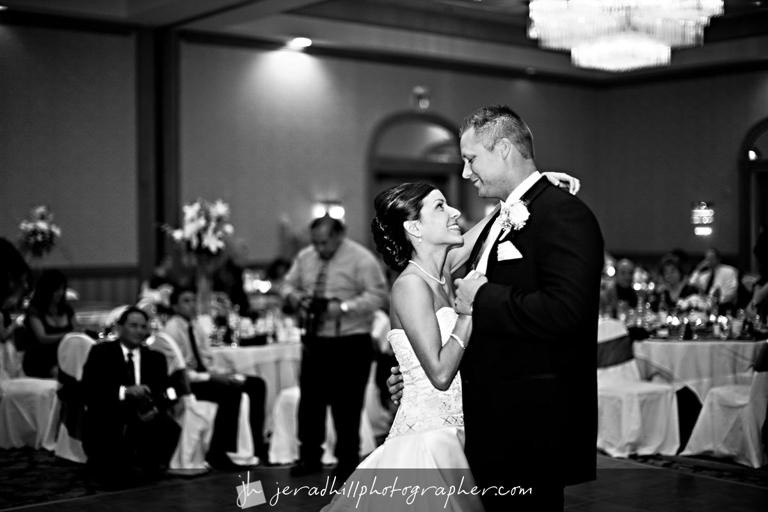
[405,260,445,286]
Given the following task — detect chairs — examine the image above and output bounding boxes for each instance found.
[680,340,767,469]
[1,340,62,451]
[269,310,391,466]
[596,319,680,459]
[146,331,259,469]
[55,332,96,464]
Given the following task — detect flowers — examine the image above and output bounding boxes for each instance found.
[20,205,62,259]
[163,198,235,275]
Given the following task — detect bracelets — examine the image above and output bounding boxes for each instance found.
[447,331,467,349]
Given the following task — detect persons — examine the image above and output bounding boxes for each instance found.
[743,276,768,339]
[321,170,582,511]
[601,258,650,343]
[453,103,606,510]
[135,264,182,325]
[164,286,276,474]
[652,257,700,338]
[686,250,741,318]
[23,269,84,379]
[278,217,389,481]
[80,307,182,494]
[0,284,27,352]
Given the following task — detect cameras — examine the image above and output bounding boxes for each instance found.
[295,295,329,334]
[137,387,178,420]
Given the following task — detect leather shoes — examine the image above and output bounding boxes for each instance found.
[204,452,257,474]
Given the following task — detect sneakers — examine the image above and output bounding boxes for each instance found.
[291,459,323,475]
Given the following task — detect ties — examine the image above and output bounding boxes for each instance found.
[127,353,135,387]
[705,271,714,295]
[187,325,206,371]
[314,258,327,301]
